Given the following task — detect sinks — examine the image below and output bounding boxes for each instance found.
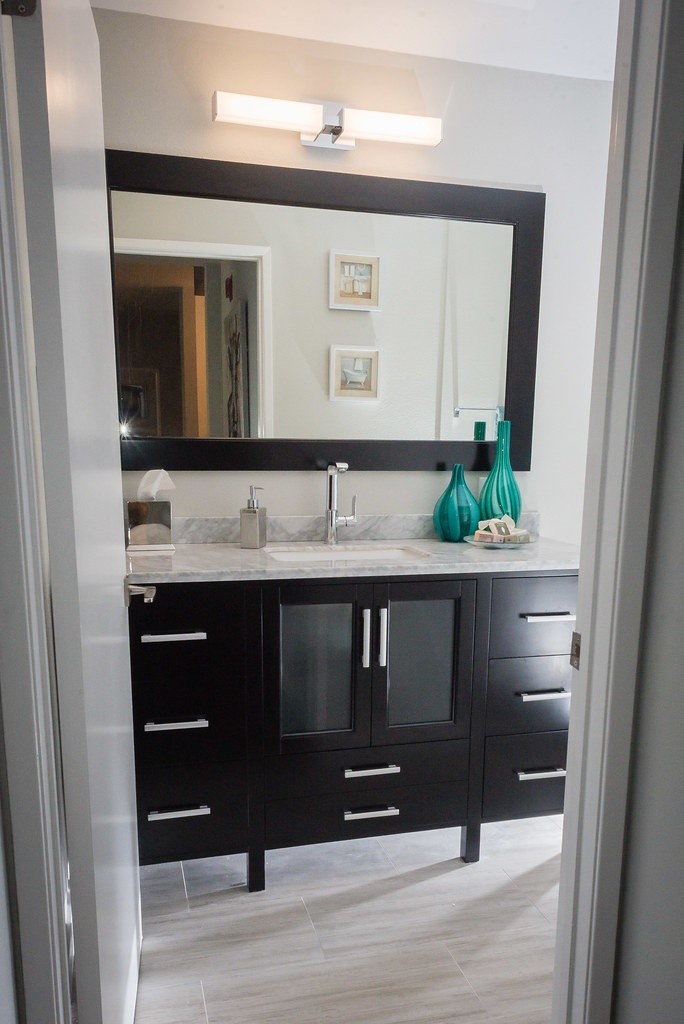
[262,542,439,562]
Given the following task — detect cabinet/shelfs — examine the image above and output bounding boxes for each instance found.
[483,568,579,822]
[127,586,249,866]
[248,572,490,891]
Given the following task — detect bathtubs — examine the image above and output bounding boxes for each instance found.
[343,369,368,386]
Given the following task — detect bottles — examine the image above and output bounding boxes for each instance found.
[474,421,486,440]
[433,464,481,543]
[477,421,522,533]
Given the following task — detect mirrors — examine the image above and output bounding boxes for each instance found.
[103,149,546,470]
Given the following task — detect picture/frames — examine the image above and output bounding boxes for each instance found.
[222,299,249,437]
[330,247,385,313]
[329,344,384,403]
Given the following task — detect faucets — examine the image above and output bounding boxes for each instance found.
[325,462,357,544]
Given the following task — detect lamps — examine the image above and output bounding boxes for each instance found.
[213,89,441,148]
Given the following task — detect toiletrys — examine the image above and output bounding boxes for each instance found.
[239,485,267,549]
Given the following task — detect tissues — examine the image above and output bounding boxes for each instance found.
[125,469,177,551]
